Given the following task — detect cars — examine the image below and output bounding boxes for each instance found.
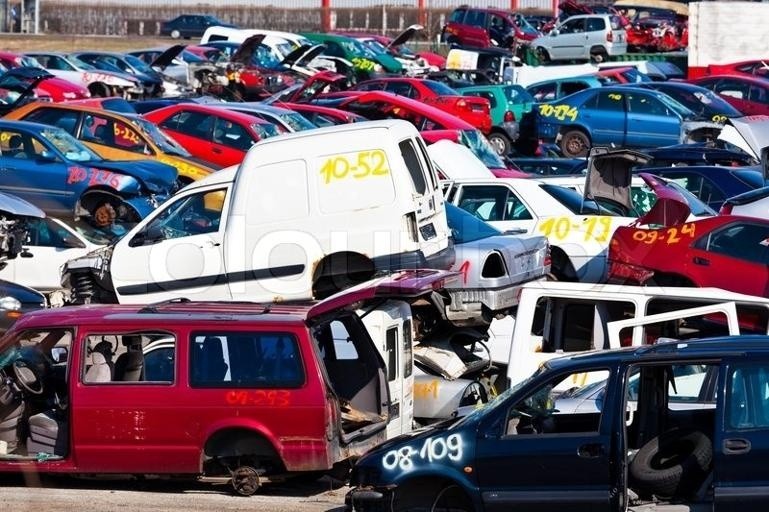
[0,192,129,374]
[346,81,490,134]
[456,82,535,157]
[680,117,769,174]
[516,62,682,98]
[22,54,145,99]
[423,138,639,282]
[538,85,711,159]
[626,13,689,53]
[140,104,285,172]
[280,93,533,181]
[1,52,91,104]
[162,14,447,96]
[671,75,769,115]
[608,171,769,348]
[413,202,552,341]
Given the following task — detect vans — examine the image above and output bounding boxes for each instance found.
[1,266,465,496]
[505,280,769,417]
[137,298,417,449]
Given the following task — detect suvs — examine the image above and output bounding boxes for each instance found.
[344,336,768,510]
[72,53,163,99]
[61,119,457,305]
[1,104,228,228]
[0,121,189,247]
[529,15,628,63]
[441,6,540,52]
